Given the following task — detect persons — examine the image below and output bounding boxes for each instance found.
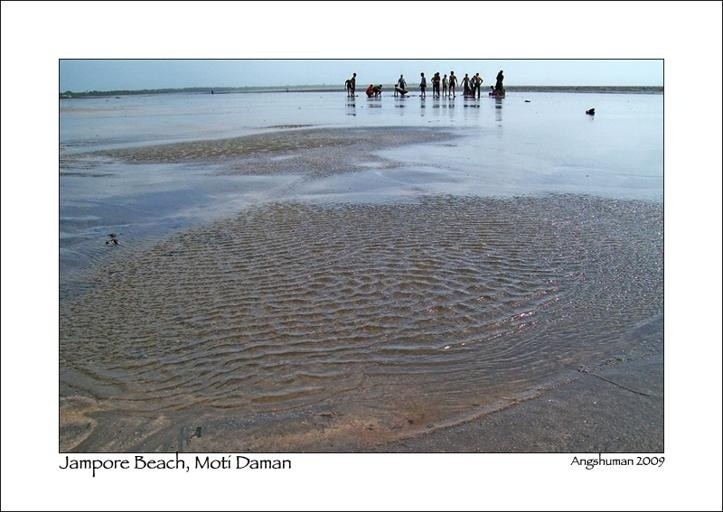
[348,72,356,96]
[418,73,426,97]
[365,83,373,98]
[393,84,407,97]
[373,83,382,97]
[430,70,505,98]
[344,79,354,97]
[397,74,406,96]
